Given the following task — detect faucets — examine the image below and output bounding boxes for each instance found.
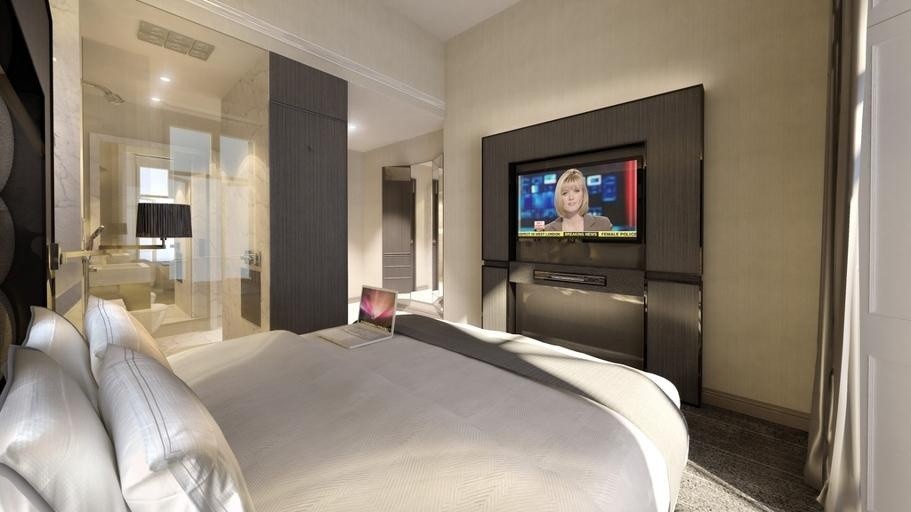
[88,223,105,244]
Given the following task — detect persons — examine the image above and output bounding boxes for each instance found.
[545,168,613,231]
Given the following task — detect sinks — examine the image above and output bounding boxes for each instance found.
[89,262,151,286]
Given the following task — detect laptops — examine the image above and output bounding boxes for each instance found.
[319,284,399,349]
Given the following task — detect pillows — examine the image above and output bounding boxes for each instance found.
[3,343,130,512]
[82,294,177,386]
[96,341,254,512]
[21,302,97,413]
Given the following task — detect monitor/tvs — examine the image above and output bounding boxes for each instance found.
[513,155,645,245]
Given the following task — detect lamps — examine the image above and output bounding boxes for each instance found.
[48,202,193,281]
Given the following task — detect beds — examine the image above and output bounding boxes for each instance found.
[167,313,691,512]
